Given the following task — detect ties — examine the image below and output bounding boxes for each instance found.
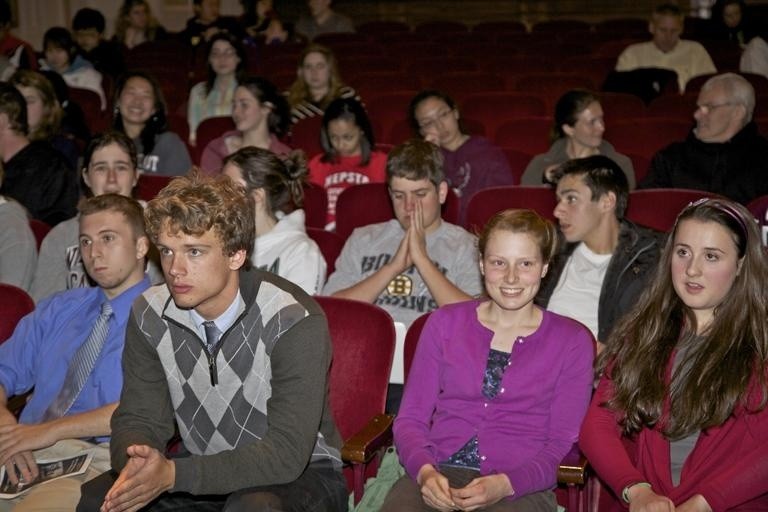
[202,321,223,357]
[39,302,115,424]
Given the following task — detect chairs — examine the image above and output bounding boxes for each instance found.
[0,16,768,512]
